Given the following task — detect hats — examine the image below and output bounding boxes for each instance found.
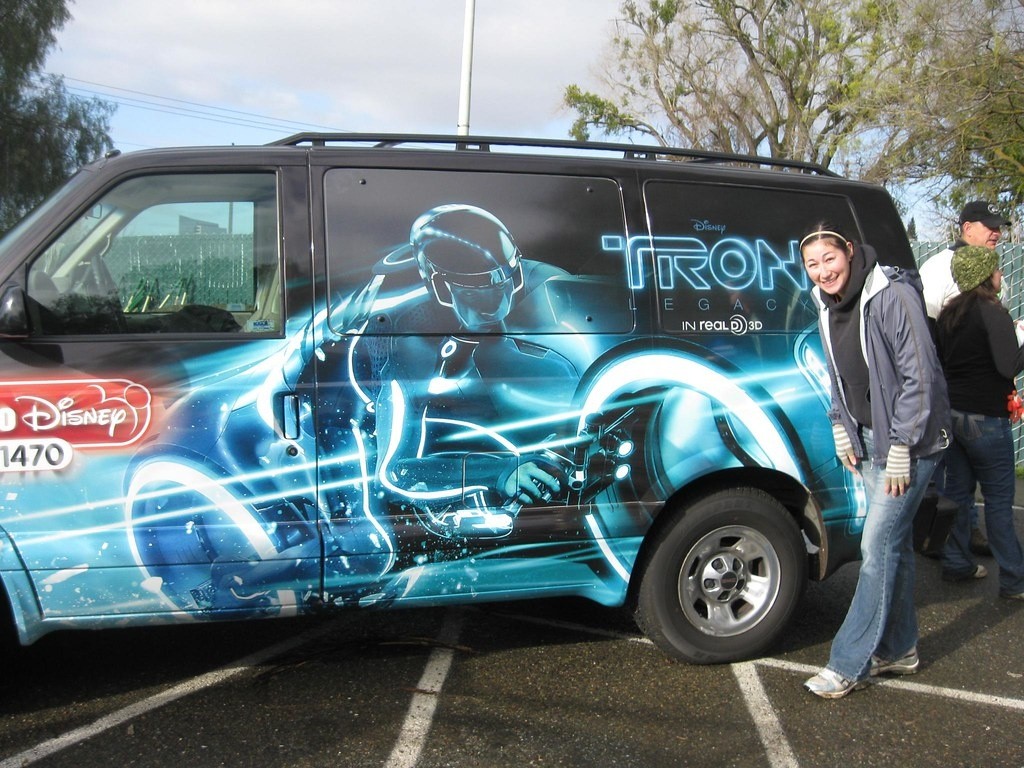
[959,200,1012,229]
[950,245,999,293]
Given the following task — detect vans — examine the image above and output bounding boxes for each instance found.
[0,131,953,694]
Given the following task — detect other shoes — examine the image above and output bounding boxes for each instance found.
[970,526,993,555]
[943,566,987,582]
[1007,593,1024,600]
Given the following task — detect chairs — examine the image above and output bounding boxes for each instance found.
[241,265,280,332]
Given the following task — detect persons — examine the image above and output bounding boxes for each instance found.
[918,200,1024,597]
[801,220,954,698]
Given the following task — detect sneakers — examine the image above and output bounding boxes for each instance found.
[868,646,920,676]
[803,666,871,700]
